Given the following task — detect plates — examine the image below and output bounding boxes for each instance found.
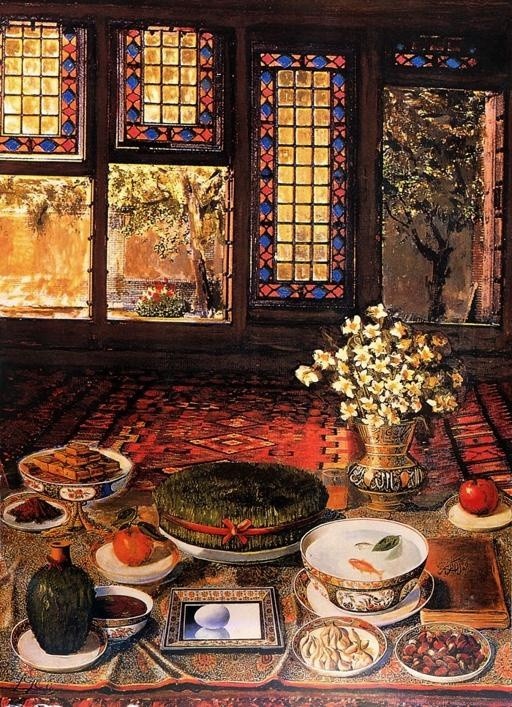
[292,568,434,627]
[89,536,180,585]
[11,617,108,673]
[395,622,491,683]
[0,491,71,531]
[291,615,388,677]
[158,526,300,567]
[443,495,512,533]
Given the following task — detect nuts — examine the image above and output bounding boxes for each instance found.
[403,629,485,677]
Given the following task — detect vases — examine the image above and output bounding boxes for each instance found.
[347,421,429,512]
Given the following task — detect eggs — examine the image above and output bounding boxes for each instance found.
[195,628,229,640]
[195,604,229,629]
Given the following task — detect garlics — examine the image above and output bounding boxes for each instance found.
[301,621,373,671]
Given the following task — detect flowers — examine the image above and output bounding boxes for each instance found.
[294,306,466,430]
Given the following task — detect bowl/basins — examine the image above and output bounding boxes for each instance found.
[93,585,154,647]
[299,518,429,614]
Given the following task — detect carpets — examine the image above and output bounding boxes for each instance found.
[0,489,511,691]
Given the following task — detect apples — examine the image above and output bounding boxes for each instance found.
[113,505,167,567]
[459,478,499,516]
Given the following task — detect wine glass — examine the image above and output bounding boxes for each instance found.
[18,447,133,536]
